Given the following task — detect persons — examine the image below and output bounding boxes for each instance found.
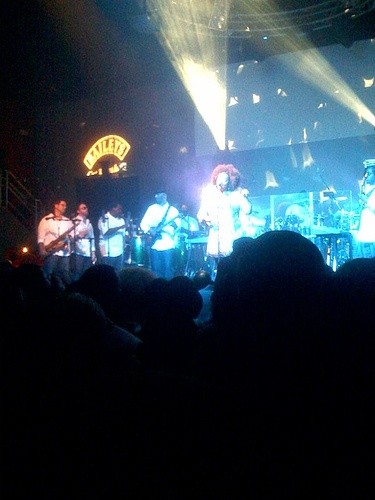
[0,165,375,500]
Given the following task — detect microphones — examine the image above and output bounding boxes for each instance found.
[46,217,57,220]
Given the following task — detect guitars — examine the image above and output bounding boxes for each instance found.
[140,212,185,248]
[23,221,81,267]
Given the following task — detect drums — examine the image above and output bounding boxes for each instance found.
[128,234,147,265]
